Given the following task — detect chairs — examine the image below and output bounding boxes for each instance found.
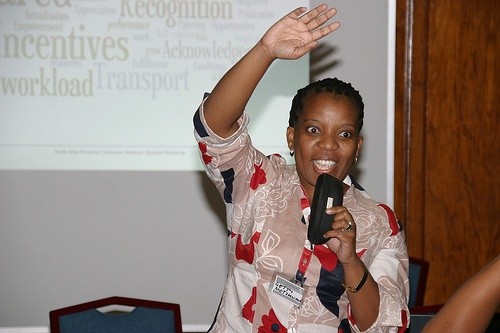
[50,296,183,333]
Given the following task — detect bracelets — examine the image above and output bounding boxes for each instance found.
[341,268,368,293]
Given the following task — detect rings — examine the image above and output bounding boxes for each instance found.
[341,221,354,232]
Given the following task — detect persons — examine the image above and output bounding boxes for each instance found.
[420,255,500,333]
[193,4,411,333]
